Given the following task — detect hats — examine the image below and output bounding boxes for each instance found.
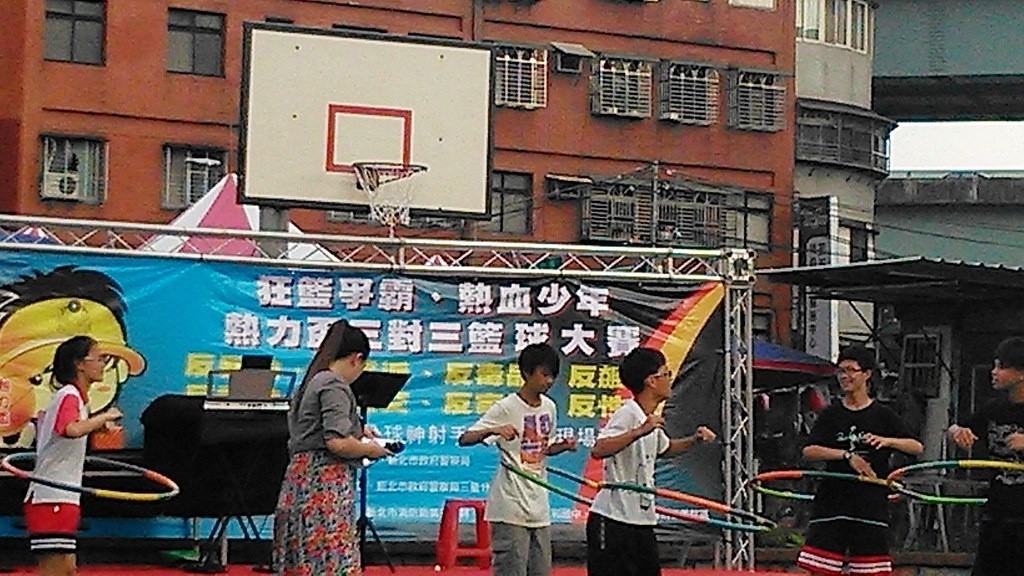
[995,336,1024,371]
[837,345,875,372]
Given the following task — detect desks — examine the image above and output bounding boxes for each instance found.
[139,395,293,576]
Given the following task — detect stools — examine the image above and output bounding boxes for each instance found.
[433,498,491,570]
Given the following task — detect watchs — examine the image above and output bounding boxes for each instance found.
[843,450,851,462]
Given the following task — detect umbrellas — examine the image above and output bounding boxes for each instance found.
[135,174,342,262]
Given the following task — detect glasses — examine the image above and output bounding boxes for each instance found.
[81,354,105,363]
[647,370,672,378]
[837,365,866,376]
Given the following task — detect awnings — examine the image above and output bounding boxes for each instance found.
[752,255,1024,326]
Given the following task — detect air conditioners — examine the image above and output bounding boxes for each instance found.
[41,172,79,197]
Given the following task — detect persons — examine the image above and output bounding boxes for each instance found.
[23,337,125,576]
[949,338,1024,576]
[797,344,924,576]
[586,347,716,575]
[273,319,395,576]
[459,343,578,576]
